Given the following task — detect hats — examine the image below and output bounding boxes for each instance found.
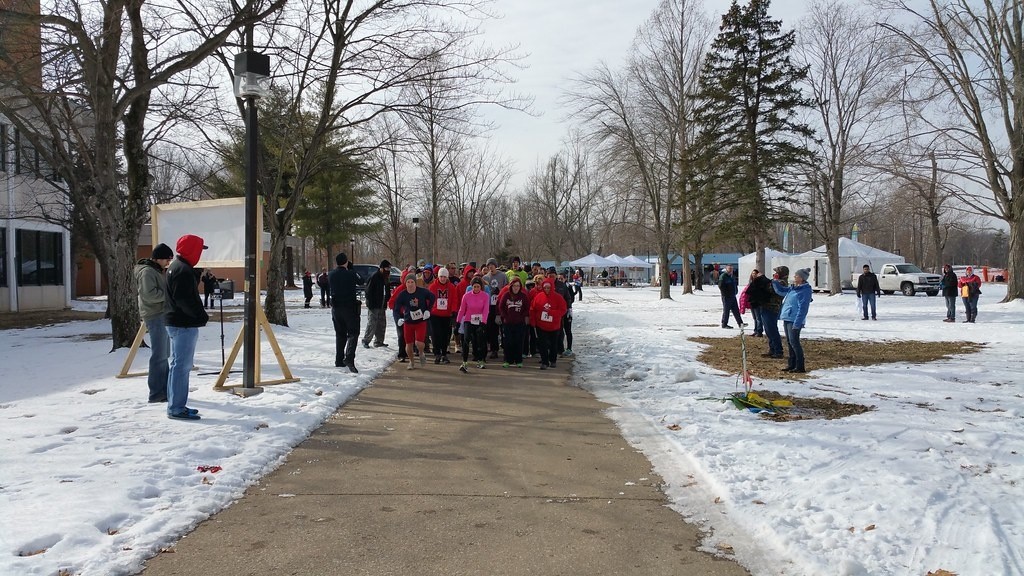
[424,268,432,273]
[486,258,497,268]
[152,244,173,259]
[202,245,208,249]
[511,257,519,265]
[306,271,311,276]
[797,268,811,280]
[405,274,415,283]
[417,259,425,267]
[543,283,551,287]
[336,253,347,265]
[438,268,449,277]
[380,260,393,267]
[546,267,557,275]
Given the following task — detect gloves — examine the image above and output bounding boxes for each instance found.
[347,261,354,269]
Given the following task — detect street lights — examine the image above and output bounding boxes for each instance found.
[350,239,355,264]
[412,218,420,269]
[232,49,272,389]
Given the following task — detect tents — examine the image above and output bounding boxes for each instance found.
[623,255,652,276]
[569,253,616,269]
[799,236,905,274]
[604,254,636,287]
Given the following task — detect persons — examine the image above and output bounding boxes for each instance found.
[620,269,624,277]
[610,276,616,286]
[936,264,982,323]
[602,269,608,286]
[362,260,393,348]
[135,243,173,403]
[857,265,880,320]
[201,268,216,309]
[573,270,582,301]
[389,257,574,373]
[317,268,330,308]
[328,253,363,372]
[302,271,315,308]
[166,235,208,420]
[579,268,584,278]
[669,269,696,286]
[717,264,811,372]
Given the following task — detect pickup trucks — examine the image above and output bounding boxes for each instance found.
[851,263,944,297]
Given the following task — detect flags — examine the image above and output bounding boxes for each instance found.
[782,224,789,251]
[852,223,858,242]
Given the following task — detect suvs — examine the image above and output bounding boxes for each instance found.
[351,264,379,289]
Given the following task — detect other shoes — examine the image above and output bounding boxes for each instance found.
[336,363,345,367]
[374,343,388,347]
[723,323,804,373]
[862,316,975,323]
[362,340,369,348]
[169,408,200,420]
[343,358,358,373]
[148,395,167,402]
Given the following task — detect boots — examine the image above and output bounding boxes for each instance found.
[304,303,312,309]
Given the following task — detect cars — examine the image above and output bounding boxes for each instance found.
[389,267,402,288]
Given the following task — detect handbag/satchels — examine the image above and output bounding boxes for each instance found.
[962,283,970,297]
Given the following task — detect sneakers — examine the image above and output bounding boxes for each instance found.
[398,336,573,373]
